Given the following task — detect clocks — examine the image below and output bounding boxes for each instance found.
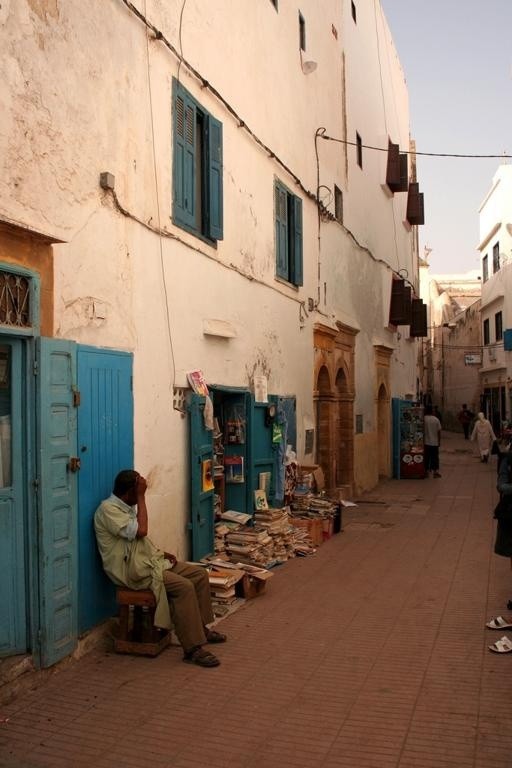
[265,403,276,427]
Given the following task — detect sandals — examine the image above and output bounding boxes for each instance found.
[486,616,512,630]
[488,636,512,654]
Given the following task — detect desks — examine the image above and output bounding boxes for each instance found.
[213,473,225,522]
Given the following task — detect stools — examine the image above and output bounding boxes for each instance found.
[113,585,172,656]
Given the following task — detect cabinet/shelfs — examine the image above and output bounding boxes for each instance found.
[399,407,425,480]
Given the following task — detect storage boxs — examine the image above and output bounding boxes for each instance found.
[213,558,274,600]
[287,516,334,547]
[299,464,325,492]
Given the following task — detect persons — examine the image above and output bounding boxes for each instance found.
[470,412,497,465]
[458,404,475,441]
[491,443,511,561]
[423,406,442,479]
[435,405,442,424]
[93,469,228,667]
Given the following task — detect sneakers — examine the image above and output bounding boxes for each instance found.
[207,631,227,643]
[183,649,220,667]
